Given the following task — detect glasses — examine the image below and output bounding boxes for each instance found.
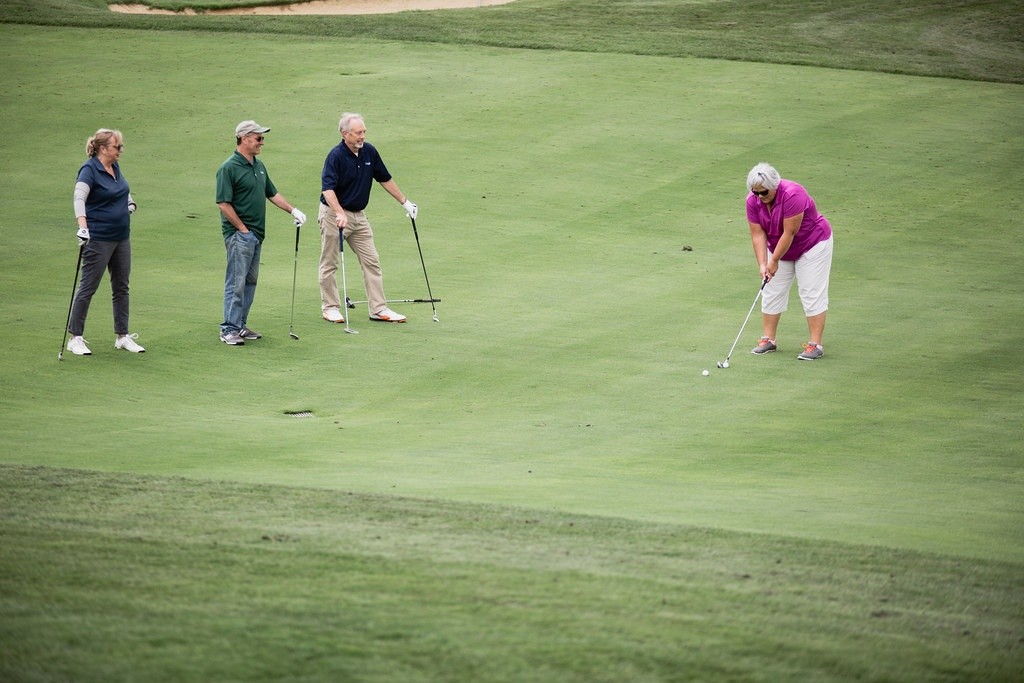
[751,188,769,196]
[255,136,265,142]
[106,144,123,150]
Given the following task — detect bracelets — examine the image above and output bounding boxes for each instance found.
[129,203,137,210]
[401,198,407,205]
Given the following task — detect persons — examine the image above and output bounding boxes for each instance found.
[66,128,146,356]
[318,113,418,323]
[745,162,833,361]
[216,120,306,345]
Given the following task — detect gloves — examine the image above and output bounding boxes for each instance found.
[128,205,136,214]
[290,208,306,227]
[402,198,418,221]
[76,227,90,246]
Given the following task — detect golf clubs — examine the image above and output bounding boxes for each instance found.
[289,222,301,339]
[58,245,84,361]
[716,276,769,368]
[339,226,359,334]
[345,296,444,308]
[411,215,439,323]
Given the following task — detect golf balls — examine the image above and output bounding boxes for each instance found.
[702,370,709,376]
[723,363,729,368]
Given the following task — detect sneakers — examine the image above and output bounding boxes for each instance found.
[369,308,407,322]
[220,330,245,345]
[238,326,262,339]
[323,307,345,323]
[67,337,92,355]
[114,333,145,353]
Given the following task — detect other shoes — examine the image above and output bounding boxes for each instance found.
[751,337,777,355]
[798,342,825,360]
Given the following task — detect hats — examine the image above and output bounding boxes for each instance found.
[235,120,271,137]
